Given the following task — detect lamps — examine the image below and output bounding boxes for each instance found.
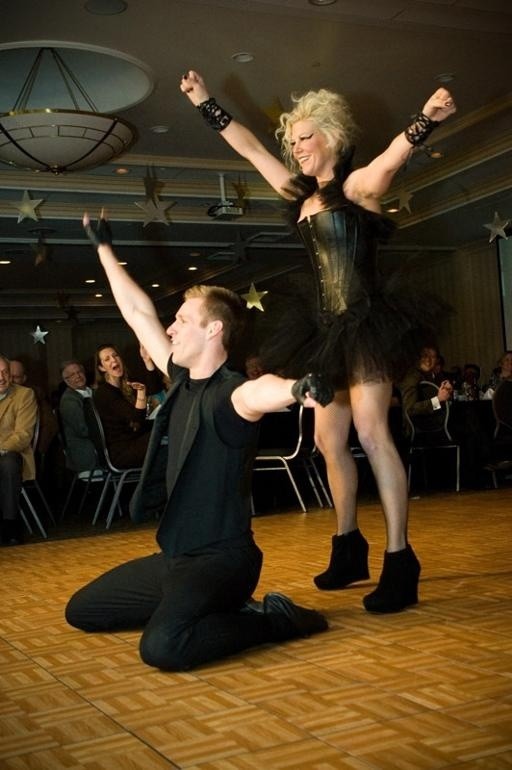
[0,47,137,176]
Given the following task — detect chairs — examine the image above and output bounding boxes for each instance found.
[249,406,333,512]
[490,381,512,488]
[82,398,144,531]
[19,420,48,542]
[294,445,328,509]
[405,380,464,494]
[55,408,124,525]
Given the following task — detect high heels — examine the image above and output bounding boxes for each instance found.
[362,543,421,614]
[313,528,371,591]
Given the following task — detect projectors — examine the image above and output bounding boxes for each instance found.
[206,204,244,223]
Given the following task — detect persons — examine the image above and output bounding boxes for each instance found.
[0,351,40,547]
[400,346,476,491]
[63,211,338,676]
[91,342,169,526]
[58,358,99,474]
[178,67,454,621]
[10,359,59,418]
[126,340,166,397]
[482,350,512,395]
[244,354,270,383]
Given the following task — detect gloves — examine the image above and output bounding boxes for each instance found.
[85,218,113,246]
[291,372,335,409]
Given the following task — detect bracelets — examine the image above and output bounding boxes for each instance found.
[193,95,235,136]
[403,111,440,150]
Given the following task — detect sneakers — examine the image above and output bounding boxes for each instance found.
[261,592,330,634]
